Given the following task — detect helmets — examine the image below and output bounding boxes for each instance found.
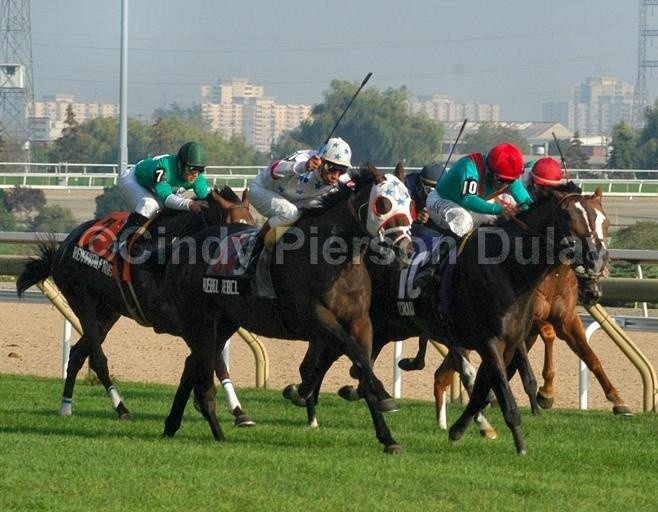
[488,143,525,181]
[421,161,446,189]
[176,141,207,167]
[531,157,563,186]
[323,137,354,167]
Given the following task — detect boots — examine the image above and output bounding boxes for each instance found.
[247,222,280,299]
[109,211,149,262]
[415,228,461,289]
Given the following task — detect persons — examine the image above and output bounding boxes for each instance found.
[107,141,208,261]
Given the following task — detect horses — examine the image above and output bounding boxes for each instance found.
[283,179,634,455]
[16,186,255,420]
[147,161,416,455]
